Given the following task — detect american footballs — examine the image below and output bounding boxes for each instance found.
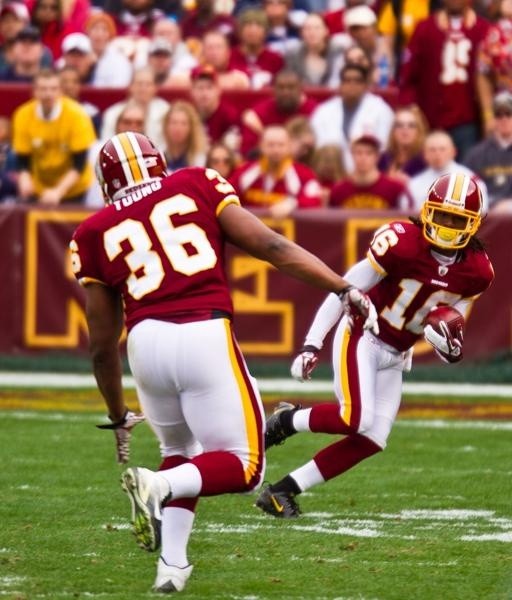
[423,306,464,339]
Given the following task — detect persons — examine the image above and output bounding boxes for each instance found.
[67,128,382,594]
[1,1,511,219]
[256,168,497,518]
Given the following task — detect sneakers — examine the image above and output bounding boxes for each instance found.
[120,464,172,554]
[258,479,302,519]
[264,402,302,451]
[151,556,194,592]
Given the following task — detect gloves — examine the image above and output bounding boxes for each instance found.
[336,283,379,336]
[107,408,145,466]
[290,351,320,382]
[422,321,465,365]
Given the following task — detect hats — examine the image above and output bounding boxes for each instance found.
[351,128,383,147]
[16,28,40,43]
[148,38,171,57]
[62,32,93,55]
[343,4,377,32]
[191,64,217,79]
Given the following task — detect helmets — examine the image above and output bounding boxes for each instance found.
[95,130,168,202]
[420,172,488,250]
